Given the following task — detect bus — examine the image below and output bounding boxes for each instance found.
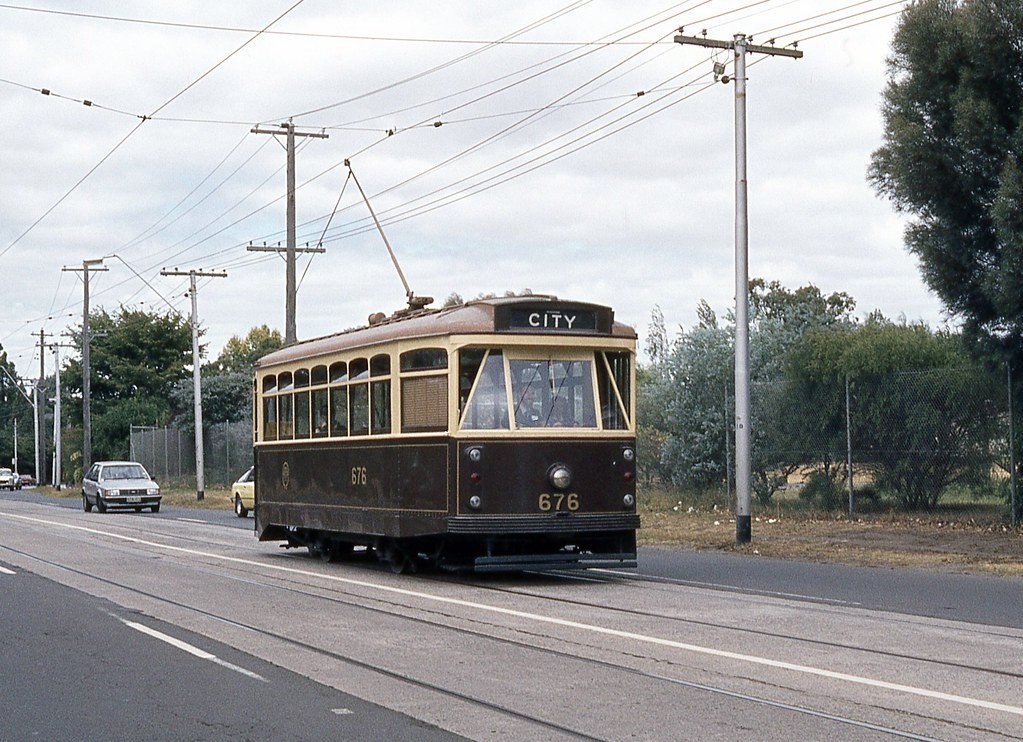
[250,166,642,575]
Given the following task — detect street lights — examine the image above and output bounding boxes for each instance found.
[102,252,207,500]
[81,332,110,473]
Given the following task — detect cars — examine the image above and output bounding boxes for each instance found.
[12,473,22,490]
[82,460,163,514]
[20,475,36,486]
[229,466,258,518]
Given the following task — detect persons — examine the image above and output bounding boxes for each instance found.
[502,385,545,426]
[315,407,347,433]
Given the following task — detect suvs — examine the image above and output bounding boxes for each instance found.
[0,467,15,491]
